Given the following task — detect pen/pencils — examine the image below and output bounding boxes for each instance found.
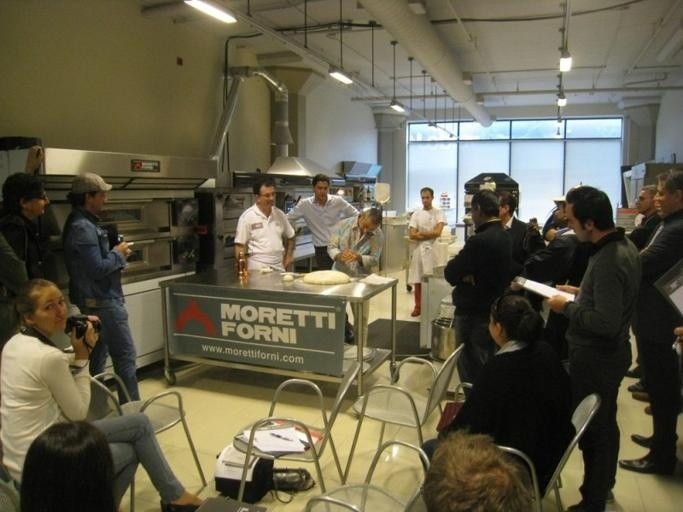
[127,242,134,246]
[565,279,569,285]
[271,432,293,442]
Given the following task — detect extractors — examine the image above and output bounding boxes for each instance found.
[265,155,346,188]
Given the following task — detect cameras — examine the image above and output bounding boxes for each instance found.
[66,315,101,338]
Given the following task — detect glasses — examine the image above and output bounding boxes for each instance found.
[37,192,48,201]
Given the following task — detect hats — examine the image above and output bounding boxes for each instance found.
[70,171,113,196]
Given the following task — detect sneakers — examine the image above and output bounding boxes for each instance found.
[645,406,652,414]
[621,365,644,378]
[411,306,422,317]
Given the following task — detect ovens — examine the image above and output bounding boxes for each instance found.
[214,181,257,264]
[21,184,204,284]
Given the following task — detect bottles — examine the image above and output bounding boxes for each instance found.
[237,251,247,278]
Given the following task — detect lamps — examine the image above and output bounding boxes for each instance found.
[181,0,461,142]
[556,41,572,139]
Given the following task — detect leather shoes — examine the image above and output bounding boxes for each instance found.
[618,455,675,476]
[627,381,654,403]
[630,434,657,449]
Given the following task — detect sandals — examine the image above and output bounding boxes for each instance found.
[160,500,200,512]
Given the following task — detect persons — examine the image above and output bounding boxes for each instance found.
[406,188,446,317]
[20,420,117,511]
[286,174,361,272]
[233,175,296,274]
[1,167,139,406]
[327,207,385,347]
[0,279,204,512]
[421,187,640,512]
[618,168,682,475]
